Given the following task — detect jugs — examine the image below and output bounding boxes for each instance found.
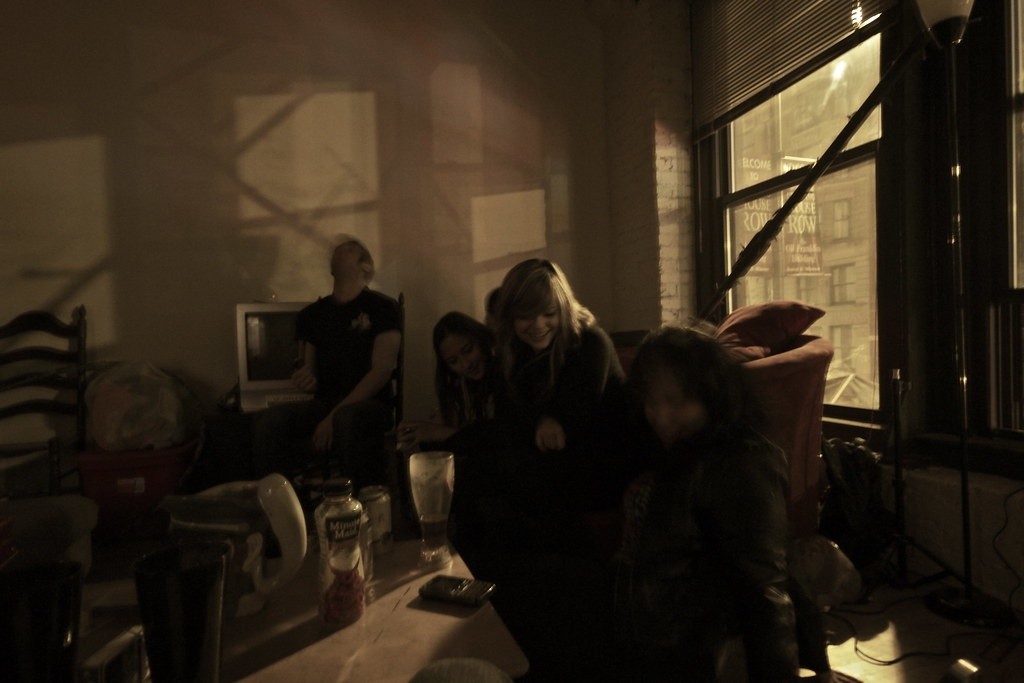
[170,472,306,614]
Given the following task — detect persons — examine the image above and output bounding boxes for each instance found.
[395,258,652,585]
[251,241,403,474]
[617,329,804,683]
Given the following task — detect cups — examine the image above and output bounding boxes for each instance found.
[135,553,225,683]
[0,560,84,683]
[409,451,454,570]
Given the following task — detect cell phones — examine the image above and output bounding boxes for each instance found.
[418,573,496,608]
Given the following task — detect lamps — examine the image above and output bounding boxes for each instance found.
[913,0,1017,627]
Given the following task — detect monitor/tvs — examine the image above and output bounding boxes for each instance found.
[236,301,320,394]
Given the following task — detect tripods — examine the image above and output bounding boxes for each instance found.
[857,367,969,608]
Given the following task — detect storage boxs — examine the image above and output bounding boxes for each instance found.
[78,435,201,542]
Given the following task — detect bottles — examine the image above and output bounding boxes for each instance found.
[314,478,366,624]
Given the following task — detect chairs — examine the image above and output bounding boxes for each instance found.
[743,332,833,536]
[316,291,412,515]
[0,303,92,498]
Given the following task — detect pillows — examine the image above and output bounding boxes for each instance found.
[719,301,826,365]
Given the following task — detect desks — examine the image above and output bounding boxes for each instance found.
[64,532,529,683]
